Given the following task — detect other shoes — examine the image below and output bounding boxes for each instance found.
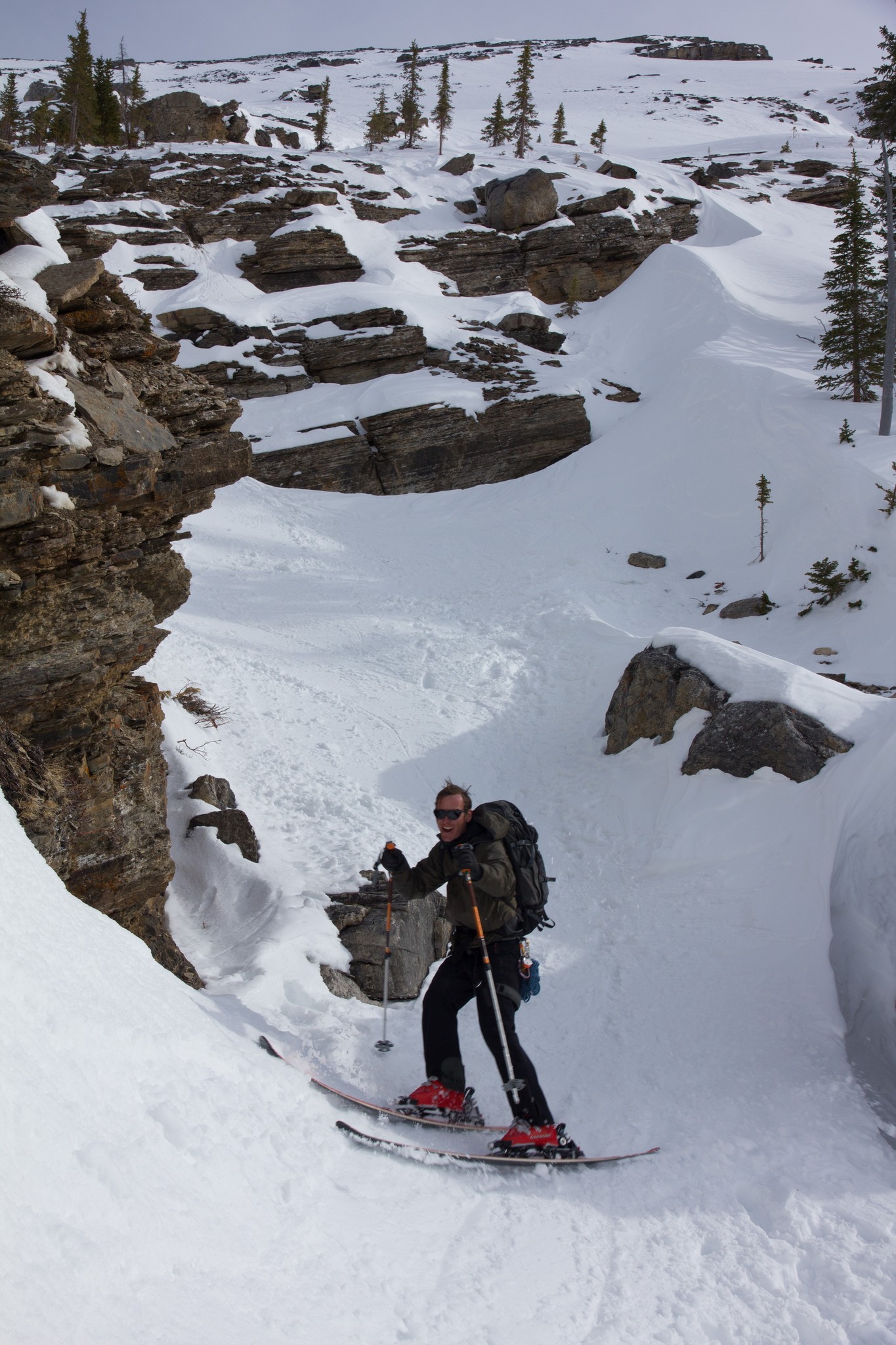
[392,1079,487,1126]
[484,1121,585,1161]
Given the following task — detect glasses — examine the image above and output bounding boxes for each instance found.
[432,808,469,820]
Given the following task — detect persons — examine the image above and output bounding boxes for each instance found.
[379,778,559,1149]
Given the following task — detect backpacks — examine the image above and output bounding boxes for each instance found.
[473,798,550,934]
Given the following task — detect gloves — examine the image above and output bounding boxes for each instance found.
[452,845,480,881]
[379,849,406,873]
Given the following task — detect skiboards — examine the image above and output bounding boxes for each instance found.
[259,1035,660,1163]
